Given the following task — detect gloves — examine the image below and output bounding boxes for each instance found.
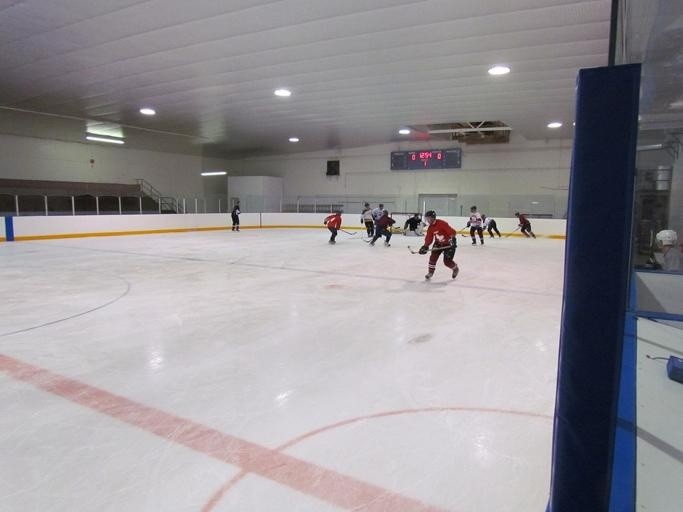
[419,246,428,254]
[449,237,456,248]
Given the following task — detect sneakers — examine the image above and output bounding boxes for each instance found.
[452,269,458,278]
[426,273,433,278]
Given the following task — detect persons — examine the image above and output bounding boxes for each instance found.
[404,213,426,236]
[466,204,485,245]
[481,214,502,238]
[655,229,683,273]
[372,204,385,224]
[514,211,536,238]
[232,200,241,232]
[324,208,343,244]
[419,210,460,279]
[360,203,375,237]
[369,209,395,247]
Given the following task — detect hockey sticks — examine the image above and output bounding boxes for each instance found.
[361,236,375,243]
[504,227,521,237]
[407,244,455,254]
[392,224,400,230]
[338,229,358,235]
[462,232,478,238]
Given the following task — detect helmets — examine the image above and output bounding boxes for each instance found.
[425,211,436,218]
